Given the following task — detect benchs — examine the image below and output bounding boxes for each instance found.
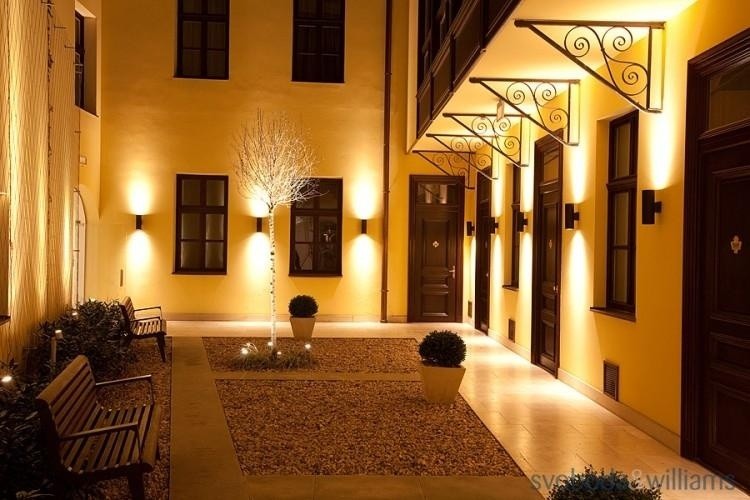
[121,296,168,364]
[35,352,164,500]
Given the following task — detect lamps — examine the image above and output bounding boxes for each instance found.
[361,218,368,234]
[255,216,263,234]
[465,220,474,236]
[516,210,529,232]
[641,189,662,225]
[564,202,580,230]
[135,213,142,230]
[49,328,69,369]
[490,217,499,235]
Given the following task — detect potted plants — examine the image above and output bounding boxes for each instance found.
[417,328,468,407]
[288,294,320,342]
